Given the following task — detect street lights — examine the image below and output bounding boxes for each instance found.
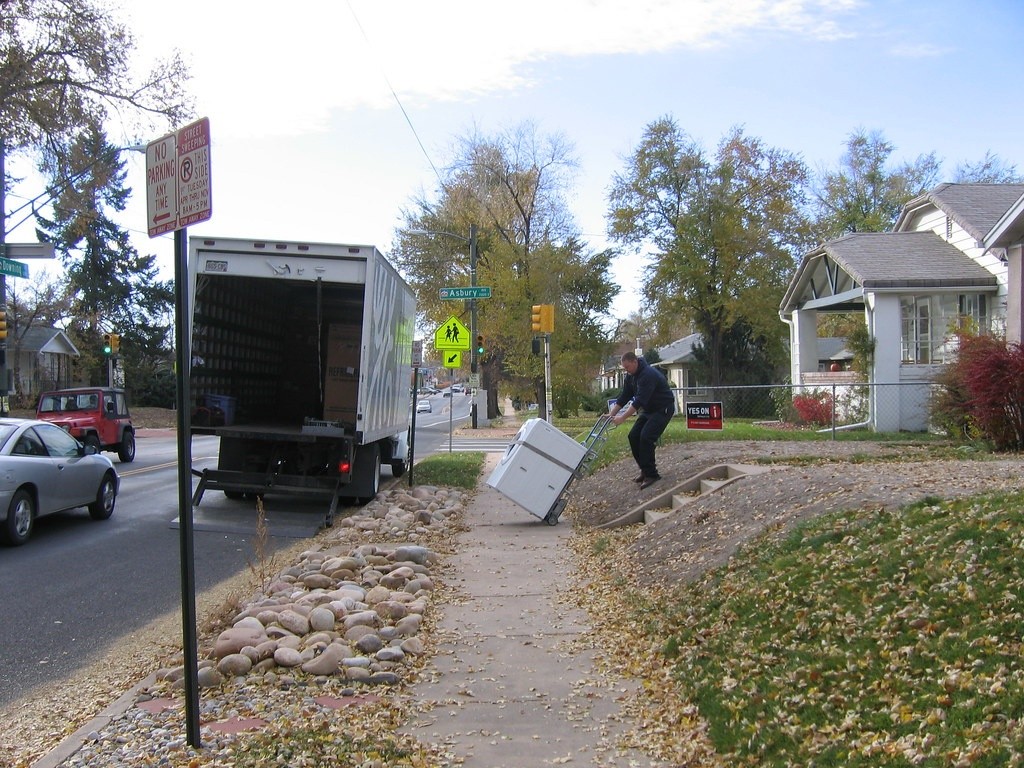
[405,223,478,430]
[0,137,149,417]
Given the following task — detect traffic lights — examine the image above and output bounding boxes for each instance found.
[0,311,9,340]
[111,333,121,354]
[530,304,543,332]
[103,333,111,355]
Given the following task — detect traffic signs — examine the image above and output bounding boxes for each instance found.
[143,134,178,238]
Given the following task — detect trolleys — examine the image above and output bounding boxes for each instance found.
[487,414,616,525]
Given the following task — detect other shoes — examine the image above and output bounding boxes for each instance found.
[641,474,660,489]
[635,472,646,483]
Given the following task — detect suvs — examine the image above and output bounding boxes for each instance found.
[35,386,135,462]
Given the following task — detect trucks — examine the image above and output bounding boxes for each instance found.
[166,234,418,538]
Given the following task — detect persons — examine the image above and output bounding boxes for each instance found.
[601,352,675,488]
[85,394,98,409]
[64,397,79,410]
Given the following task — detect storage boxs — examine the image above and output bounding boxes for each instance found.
[323,322,361,425]
[204,393,237,426]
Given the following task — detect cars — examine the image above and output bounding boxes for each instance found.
[416,400,432,413]
[441,382,476,397]
[0,417,121,545]
[416,385,441,394]
